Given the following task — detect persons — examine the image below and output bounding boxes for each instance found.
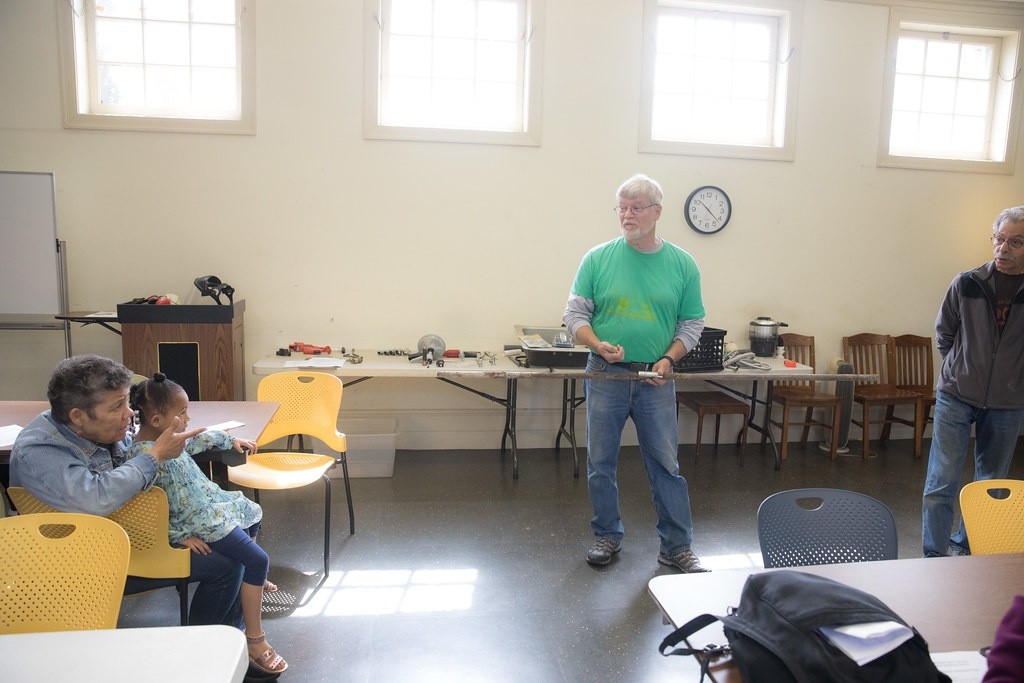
[10,354,245,630]
[921,205,1024,557]
[130,371,289,673]
[562,174,712,573]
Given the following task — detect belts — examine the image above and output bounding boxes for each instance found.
[600,355,654,372]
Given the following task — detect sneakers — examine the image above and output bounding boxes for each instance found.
[656,548,712,572]
[586,539,622,565]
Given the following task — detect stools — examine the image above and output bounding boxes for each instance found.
[675,391,749,454]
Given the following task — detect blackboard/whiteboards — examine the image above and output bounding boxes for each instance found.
[0,170,61,317]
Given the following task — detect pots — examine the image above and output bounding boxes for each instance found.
[749,316,788,357]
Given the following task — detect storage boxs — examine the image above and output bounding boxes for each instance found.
[311,414,401,477]
[673,328,727,373]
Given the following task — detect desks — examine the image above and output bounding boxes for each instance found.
[253,350,551,487]
[0,624,250,683]
[0,399,280,491]
[649,551,1024,683]
[554,342,807,481]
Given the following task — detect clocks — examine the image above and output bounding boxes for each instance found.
[683,185,732,233]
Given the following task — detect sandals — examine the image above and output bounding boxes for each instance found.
[264,580,278,593]
[244,629,288,673]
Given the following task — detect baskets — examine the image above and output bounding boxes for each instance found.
[673,327,728,373]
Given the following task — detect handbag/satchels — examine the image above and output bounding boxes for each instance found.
[721,570,953,683]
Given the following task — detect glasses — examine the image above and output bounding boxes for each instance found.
[990,234,1024,250]
[614,204,658,214]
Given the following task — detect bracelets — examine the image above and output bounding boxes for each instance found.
[658,356,674,367]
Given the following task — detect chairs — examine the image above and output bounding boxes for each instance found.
[0,371,360,636]
[959,478,1024,555]
[757,487,897,571]
[883,336,945,456]
[759,332,849,461]
[843,333,924,458]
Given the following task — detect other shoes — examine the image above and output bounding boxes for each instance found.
[245,665,281,681]
